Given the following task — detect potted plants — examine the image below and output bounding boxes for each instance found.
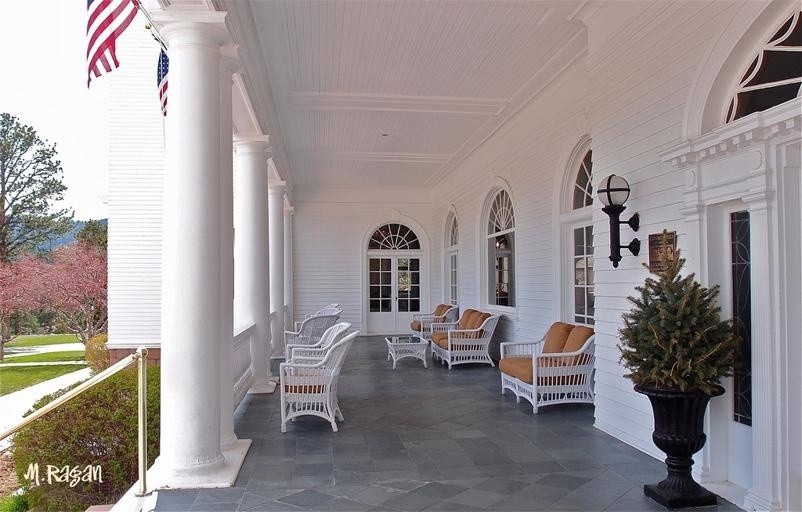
[616,229,746,508]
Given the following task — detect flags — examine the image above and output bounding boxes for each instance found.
[86,0,141,88]
[157,51,168,116]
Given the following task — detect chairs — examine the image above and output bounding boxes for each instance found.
[279,304,360,433]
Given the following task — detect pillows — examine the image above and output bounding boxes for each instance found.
[560,326,594,365]
[459,309,491,338]
[436,304,444,316]
[543,322,574,362]
[441,305,453,323]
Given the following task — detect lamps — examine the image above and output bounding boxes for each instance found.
[597,175,640,268]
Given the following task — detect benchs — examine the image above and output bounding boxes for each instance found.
[431,307,502,370]
[499,321,595,414]
[409,304,459,342]
